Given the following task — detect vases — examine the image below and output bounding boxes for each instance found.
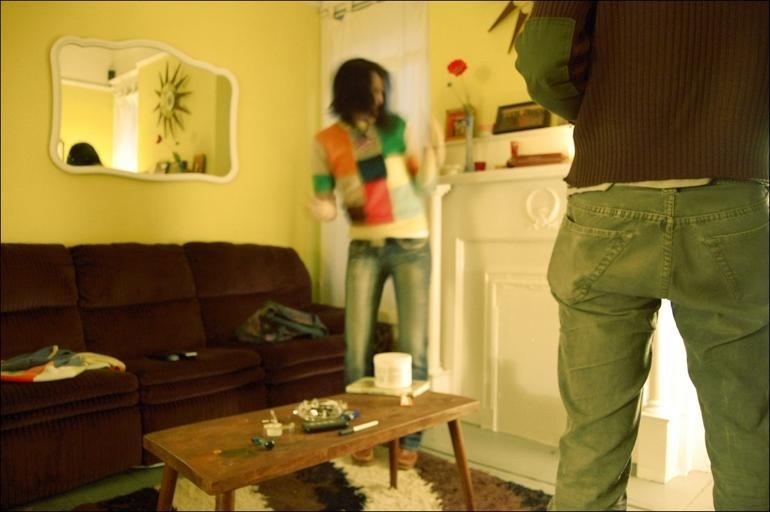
[463,112,478,172]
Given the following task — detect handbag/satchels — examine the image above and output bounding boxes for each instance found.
[232,298,329,346]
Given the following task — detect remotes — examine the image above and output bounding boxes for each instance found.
[179,351,199,360]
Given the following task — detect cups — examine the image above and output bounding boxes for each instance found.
[372,350,413,391]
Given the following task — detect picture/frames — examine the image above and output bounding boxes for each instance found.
[490,100,552,135]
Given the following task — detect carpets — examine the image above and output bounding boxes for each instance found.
[70,446,554,512]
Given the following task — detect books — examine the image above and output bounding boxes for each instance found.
[345,376,430,398]
[508,152,568,165]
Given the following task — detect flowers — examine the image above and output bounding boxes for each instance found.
[441,58,479,112]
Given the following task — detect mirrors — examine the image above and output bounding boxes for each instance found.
[49,34,240,187]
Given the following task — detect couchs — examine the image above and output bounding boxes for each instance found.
[0,238,396,510]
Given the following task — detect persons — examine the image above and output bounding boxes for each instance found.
[511,1,770,512]
[310,57,434,473]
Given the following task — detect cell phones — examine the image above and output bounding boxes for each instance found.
[302,417,348,430]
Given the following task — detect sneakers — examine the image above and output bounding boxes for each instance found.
[352,444,375,463]
[395,447,418,470]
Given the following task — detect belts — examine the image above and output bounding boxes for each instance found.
[349,236,411,250]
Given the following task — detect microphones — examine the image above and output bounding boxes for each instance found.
[146,350,181,362]
